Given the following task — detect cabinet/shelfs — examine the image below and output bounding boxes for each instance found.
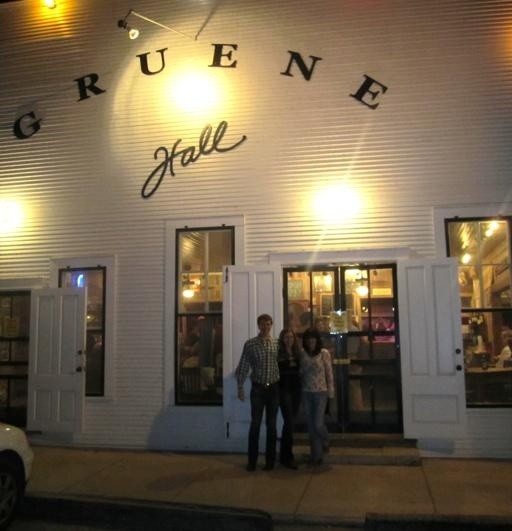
[353,294,396,342]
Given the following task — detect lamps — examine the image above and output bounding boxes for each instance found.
[114,4,197,48]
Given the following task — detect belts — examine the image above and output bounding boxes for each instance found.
[253,382,278,388]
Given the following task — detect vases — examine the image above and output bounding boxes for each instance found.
[475,335,488,356]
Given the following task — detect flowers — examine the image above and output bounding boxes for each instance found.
[467,311,487,335]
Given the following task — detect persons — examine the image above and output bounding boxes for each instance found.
[184,316,216,392]
[493,330,512,368]
[276,327,302,470]
[235,314,280,471]
[288,312,364,416]
[299,328,335,466]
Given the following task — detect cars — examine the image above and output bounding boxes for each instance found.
[0,421,36,531]
